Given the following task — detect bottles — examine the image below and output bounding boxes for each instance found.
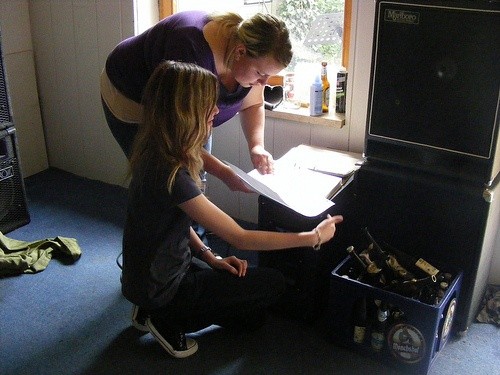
[310,73,323,117]
[336,67,348,113]
[319,61,330,112]
[339,224,453,354]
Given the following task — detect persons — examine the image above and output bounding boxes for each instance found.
[99,10,293,260]
[122,61,344,358]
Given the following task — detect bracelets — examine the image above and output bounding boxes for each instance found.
[313,228,321,250]
[198,246,211,260]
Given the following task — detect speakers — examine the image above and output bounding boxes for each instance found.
[355,161,500,337]
[0,32,31,234]
[364,0,500,185]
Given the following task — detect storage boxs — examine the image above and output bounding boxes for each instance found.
[328,252,464,375]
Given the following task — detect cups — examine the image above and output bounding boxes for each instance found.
[284,74,305,110]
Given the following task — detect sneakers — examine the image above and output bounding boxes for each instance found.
[131,304,150,331]
[145,316,200,357]
